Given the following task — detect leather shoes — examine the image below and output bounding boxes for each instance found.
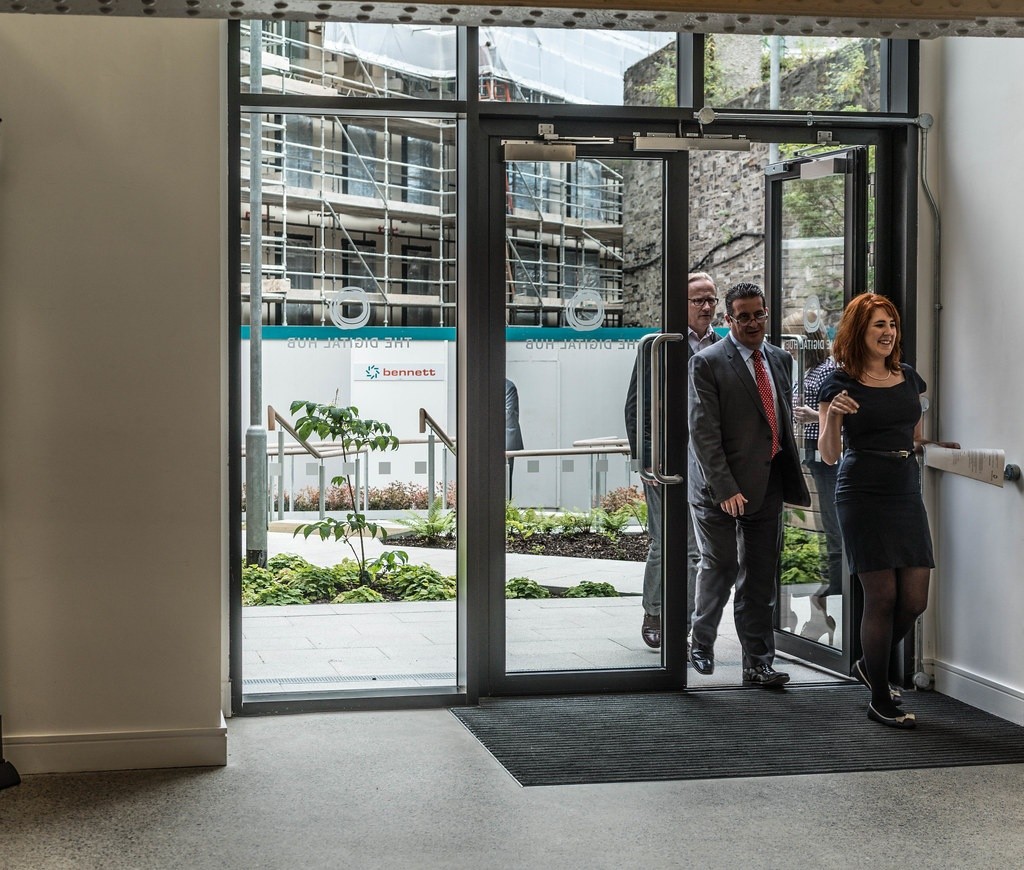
[642,613,661,648]
[687,633,714,674]
[742,664,790,687]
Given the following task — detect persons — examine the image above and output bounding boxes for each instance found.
[815,294,960,726]
[506,376,524,500]
[624,272,845,688]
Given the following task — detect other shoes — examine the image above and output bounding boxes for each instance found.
[867,702,915,729]
[851,660,903,706]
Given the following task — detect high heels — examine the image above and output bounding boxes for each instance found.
[800,615,835,645]
[780,611,797,633]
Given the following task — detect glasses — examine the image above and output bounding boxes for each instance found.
[730,310,768,326]
[688,298,719,308]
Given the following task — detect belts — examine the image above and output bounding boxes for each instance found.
[862,449,913,458]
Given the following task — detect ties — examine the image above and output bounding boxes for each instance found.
[750,350,779,458]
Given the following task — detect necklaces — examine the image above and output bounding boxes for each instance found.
[860,366,893,382]
